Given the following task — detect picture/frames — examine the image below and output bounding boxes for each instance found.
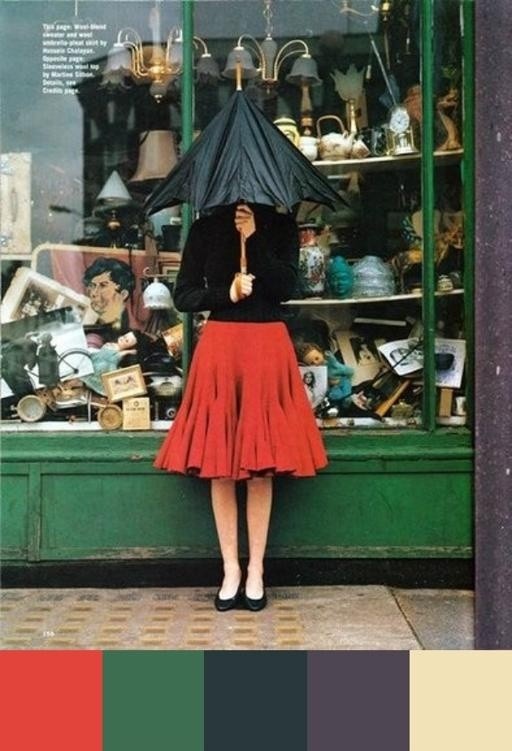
[1,266,100,326]
[101,365,148,403]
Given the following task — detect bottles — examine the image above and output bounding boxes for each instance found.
[299,117,316,138]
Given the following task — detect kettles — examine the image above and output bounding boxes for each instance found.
[437,273,454,293]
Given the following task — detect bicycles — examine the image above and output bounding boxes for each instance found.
[23,343,92,389]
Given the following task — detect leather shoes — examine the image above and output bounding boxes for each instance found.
[215,570,245,611]
[245,588,267,611]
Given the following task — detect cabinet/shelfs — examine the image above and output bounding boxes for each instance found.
[281,149,463,305]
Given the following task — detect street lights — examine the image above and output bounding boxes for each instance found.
[46,203,120,242]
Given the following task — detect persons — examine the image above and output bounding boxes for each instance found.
[82,256,137,330]
[301,367,318,405]
[84,332,137,399]
[294,339,354,399]
[152,203,330,612]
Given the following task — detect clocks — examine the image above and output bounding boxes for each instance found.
[387,103,420,156]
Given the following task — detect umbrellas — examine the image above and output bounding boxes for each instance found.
[138,58,351,274]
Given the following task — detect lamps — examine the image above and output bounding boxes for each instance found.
[102,1,218,103]
[98,170,133,248]
[222,1,324,90]
[126,130,179,190]
[329,63,366,138]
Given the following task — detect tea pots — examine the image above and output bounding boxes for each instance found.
[272,115,299,148]
[315,115,356,159]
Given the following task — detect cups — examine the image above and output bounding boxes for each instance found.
[297,136,317,162]
